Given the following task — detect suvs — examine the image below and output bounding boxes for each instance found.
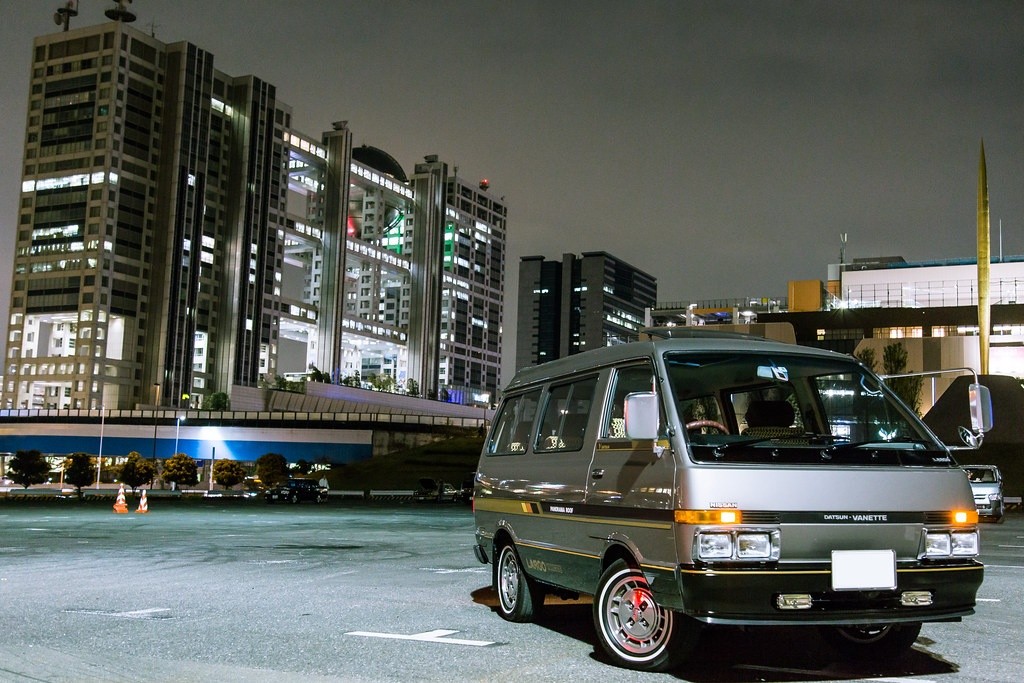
[412,479,440,505]
[958,464,1008,524]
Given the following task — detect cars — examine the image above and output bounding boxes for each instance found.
[265,479,330,506]
[439,483,472,506]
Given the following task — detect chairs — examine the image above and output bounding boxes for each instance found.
[558,414,589,439]
[745,400,795,429]
[514,422,552,445]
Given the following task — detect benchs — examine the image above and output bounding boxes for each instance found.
[328,491,364,497]
[370,490,414,499]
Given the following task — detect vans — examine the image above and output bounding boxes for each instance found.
[472,324,985,674]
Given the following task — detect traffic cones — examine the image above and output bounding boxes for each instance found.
[112,482,129,514]
[133,490,152,513]
